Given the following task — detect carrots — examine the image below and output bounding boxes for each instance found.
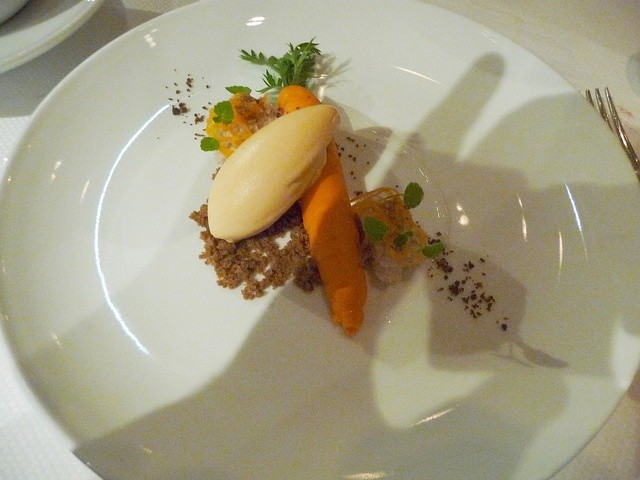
[236,36,367,336]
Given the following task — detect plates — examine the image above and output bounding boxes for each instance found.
[2,0,101,73]
[1,0,640,480]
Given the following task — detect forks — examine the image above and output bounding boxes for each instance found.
[583,88,640,184]
